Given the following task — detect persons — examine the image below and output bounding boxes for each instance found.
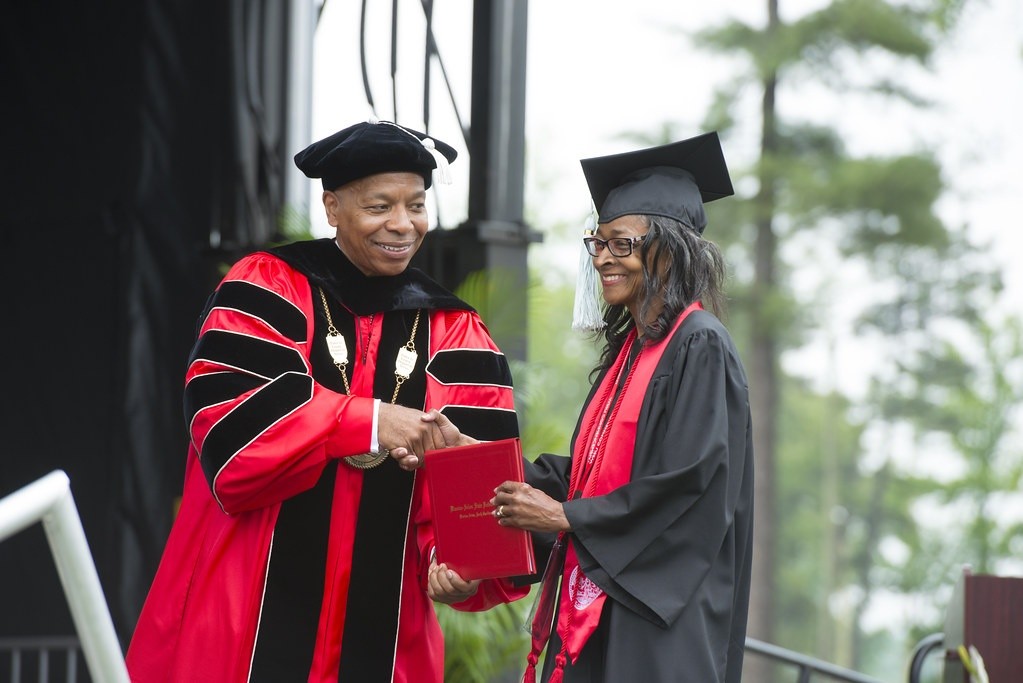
[124,119,533,682]
[392,132,753,683]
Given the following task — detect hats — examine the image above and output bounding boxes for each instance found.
[572,131,734,330]
[294,116,457,193]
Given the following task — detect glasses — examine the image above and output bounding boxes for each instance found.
[583,231,659,258]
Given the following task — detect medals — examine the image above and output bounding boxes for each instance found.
[343,444,389,469]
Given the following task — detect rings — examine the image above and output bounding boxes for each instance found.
[497,505,507,517]
